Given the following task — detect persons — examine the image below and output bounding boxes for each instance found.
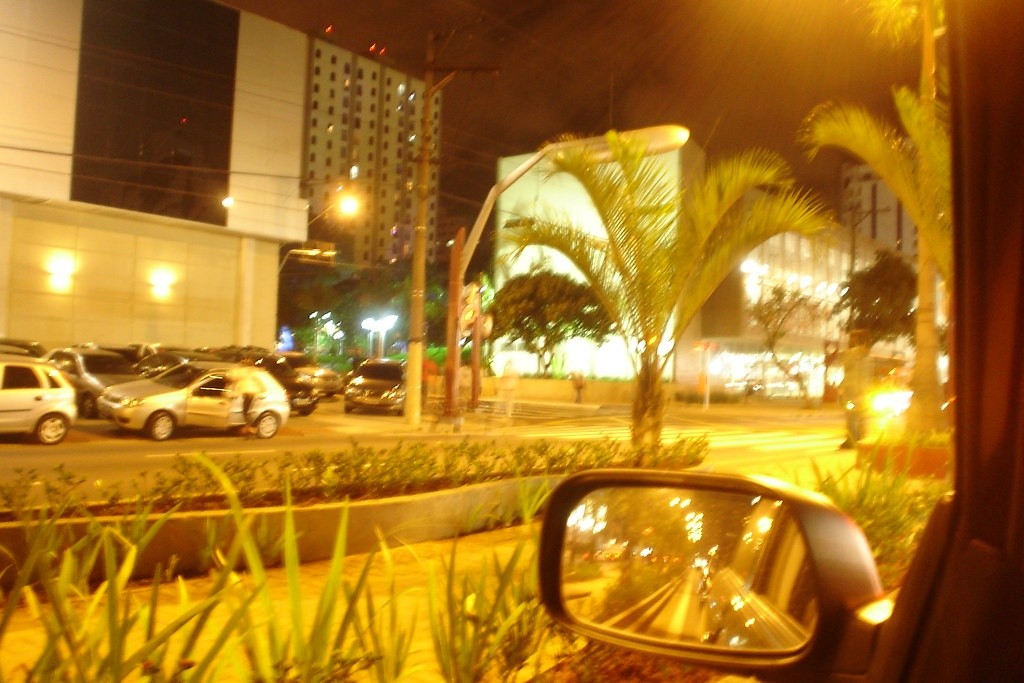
[421,356,473,433]
[570,370,587,404]
[352,347,364,372]
[742,378,757,406]
[496,360,517,420]
[837,366,871,449]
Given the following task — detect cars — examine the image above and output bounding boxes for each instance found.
[279,351,343,400]
[0,353,80,449]
[41,347,144,420]
[98,360,291,441]
[98,340,322,415]
[340,356,406,417]
[0,335,48,359]
